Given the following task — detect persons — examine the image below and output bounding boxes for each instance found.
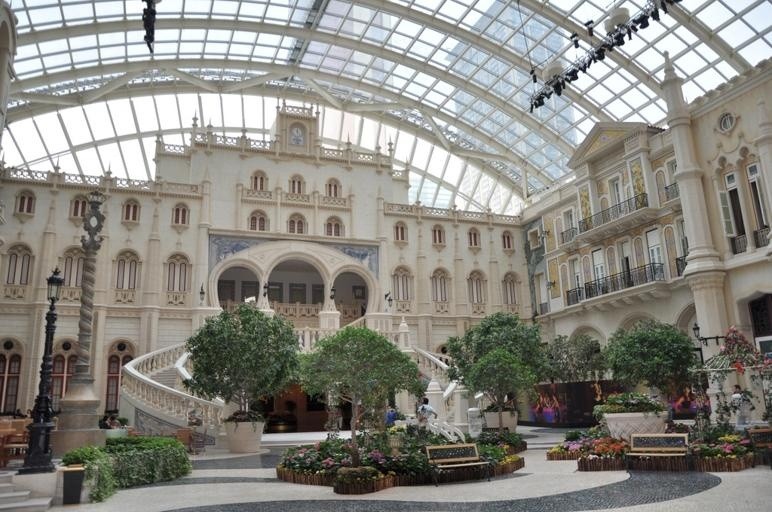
[99,415,111,429]
[336,406,343,429]
[387,401,398,427]
[536,392,560,417]
[727,385,742,412]
[110,415,121,427]
[14,409,27,418]
[675,386,691,417]
[418,397,437,425]
[27,409,31,419]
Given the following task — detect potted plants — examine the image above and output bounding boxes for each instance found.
[593,391,669,441]
[482,402,517,433]
[54,439,119,505]
[225,409,265,453]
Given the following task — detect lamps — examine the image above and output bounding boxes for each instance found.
[142,0,157,62]
[385,292,392,307]
[330,286,336,299]
[692,323,707,344]
[546,281,555,289]
[516,0,682,113]
[200,282,205,301]
[541,230,549,237]
[263,283,267,296]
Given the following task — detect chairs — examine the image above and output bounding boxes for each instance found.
[122,426,207,455]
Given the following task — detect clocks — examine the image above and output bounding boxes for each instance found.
[293,126,303,137]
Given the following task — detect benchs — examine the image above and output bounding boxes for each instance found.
[626,433,689,472]
[0,413,34,468]
[424,443,491,487]
[744,427,772,470]
